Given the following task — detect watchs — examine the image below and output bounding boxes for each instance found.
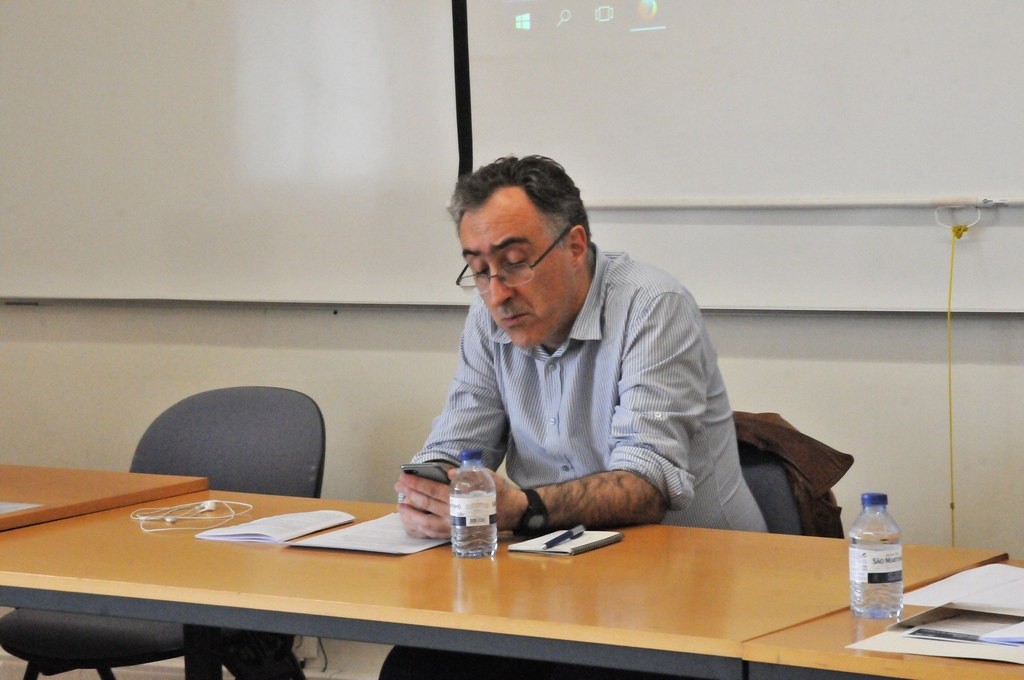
[513,489,548,537]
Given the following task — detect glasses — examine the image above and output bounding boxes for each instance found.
[454,226,570,298]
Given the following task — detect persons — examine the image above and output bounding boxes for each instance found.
[377,155,767,680]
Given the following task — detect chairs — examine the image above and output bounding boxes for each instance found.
[0,384,325,680]
[733,410,854,539]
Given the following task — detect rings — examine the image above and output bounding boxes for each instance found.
[397,492,404,504]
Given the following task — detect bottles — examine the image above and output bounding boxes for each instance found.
[847,493,903,619]
[448,448,498,558]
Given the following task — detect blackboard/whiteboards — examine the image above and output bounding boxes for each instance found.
[1,1,1023,317]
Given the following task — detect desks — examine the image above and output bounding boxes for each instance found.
[0,488,1009,680]
[743,556,1024,680]
[0,464,208,532]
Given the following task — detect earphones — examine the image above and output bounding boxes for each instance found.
[196,501,209,510]
[199,501,216,514]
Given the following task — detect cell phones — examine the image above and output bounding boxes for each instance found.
[401,464,450,487]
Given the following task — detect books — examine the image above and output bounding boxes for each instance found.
[508,529,623,556]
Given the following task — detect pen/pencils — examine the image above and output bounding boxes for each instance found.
[540,522,588,551]
